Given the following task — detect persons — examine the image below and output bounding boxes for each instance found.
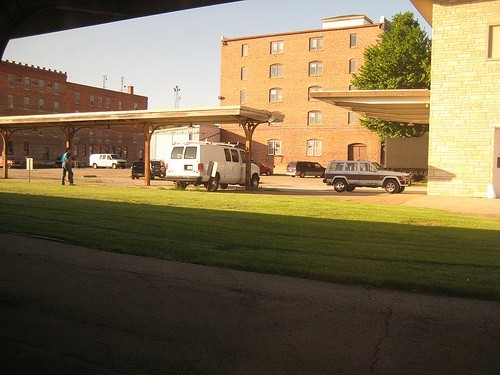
[61,148,76,185]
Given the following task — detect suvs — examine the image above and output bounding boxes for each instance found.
[164,138,260,192]
[287,161,327,179]
[323,158,419,194]
[150,159,167,178]
[131,161,156,180]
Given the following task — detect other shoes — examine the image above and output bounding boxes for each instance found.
[62,182,64,185]
[69,183,74,185]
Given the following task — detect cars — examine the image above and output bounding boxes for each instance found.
[254,161,273,176]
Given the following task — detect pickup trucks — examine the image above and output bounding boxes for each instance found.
[89,153,127,170]
